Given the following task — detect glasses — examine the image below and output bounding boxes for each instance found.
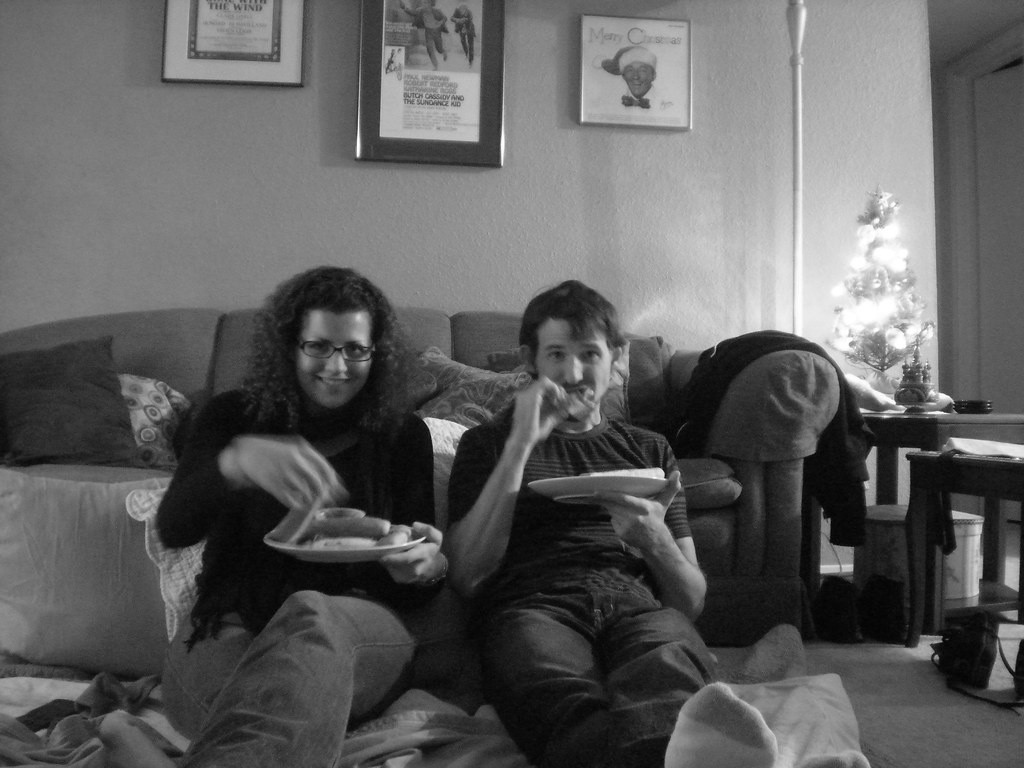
[301,339,376,362]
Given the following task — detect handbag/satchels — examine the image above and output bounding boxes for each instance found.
[931,607,1024,707]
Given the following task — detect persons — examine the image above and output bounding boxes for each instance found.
[153,267,448,768]
[386,48,404,79]
[400,0,477,71]
[444,280,716,768]
[620,46,663,108]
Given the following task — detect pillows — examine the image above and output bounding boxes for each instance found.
[406,346,528,427]
[626,335,666,423]
[479,336,632,424]
[1,334,135,467]
[1,471,194,675]
[115,374,193,467]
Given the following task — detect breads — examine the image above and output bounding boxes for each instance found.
[269,484,412,548]
[579,468,665,479]
[562,388,602,424]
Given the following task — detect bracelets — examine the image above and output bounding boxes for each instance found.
[424,560,448,585]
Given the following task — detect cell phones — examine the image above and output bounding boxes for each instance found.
[16,697,83,733]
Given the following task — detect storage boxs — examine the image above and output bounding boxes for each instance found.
[853,503,983,599]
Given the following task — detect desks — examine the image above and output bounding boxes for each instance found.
[802,411,1024,646]
[906,447,1024,701]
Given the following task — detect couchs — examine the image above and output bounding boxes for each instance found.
[1,308,838,647]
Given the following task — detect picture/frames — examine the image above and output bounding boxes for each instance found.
[355,0,505,168]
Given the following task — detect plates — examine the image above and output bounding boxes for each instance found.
[953,400,994,414]
[526,476,671,504]
[264,526,428,561]
